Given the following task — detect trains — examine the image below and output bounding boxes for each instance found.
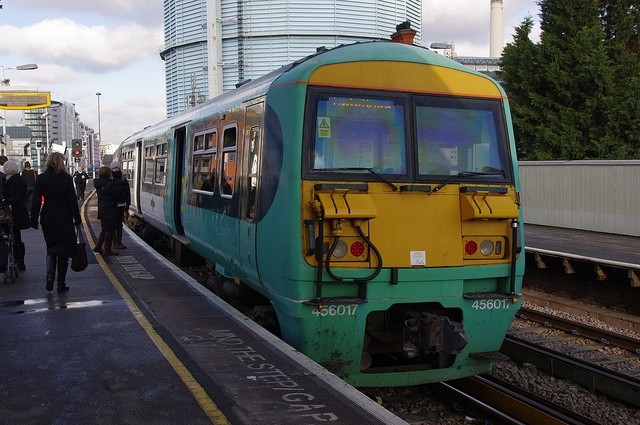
[112,38,527,388]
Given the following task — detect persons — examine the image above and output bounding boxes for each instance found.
[24,160,32,216]
[111,158,131,248]
[93,166,119,256]
[201,168,232,195]
[0,156,8,280]
[32,151,81,292]
[3,160,31,272]
[81,169,88,199]
[74,171,82,200]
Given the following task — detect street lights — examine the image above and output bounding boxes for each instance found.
[1,64,38,156]
[96,93,101,168]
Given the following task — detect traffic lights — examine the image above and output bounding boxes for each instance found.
[72,139,82,156]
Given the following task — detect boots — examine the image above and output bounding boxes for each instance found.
[102,241,119,256]
[57,261,70,293]
[46,255,57,291]
[112,230,126,249]
[116,225,122,242]
[93,241,103,255]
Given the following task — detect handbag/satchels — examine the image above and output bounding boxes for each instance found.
[71,225,89,272]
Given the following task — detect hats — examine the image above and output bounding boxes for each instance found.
[110,161,121,170]
[3,160,19,176]
